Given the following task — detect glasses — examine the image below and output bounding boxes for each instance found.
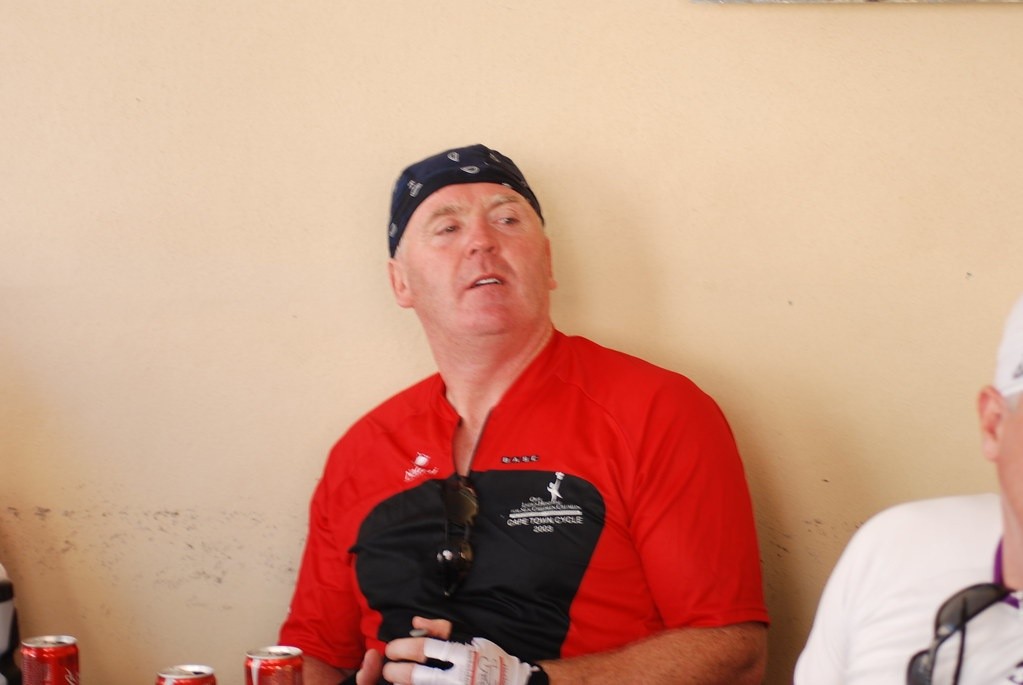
[906,583,1016,685]
[432,474,480,596]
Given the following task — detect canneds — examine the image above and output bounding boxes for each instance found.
[156,664,217,685]
[19,634,80,685]
[244,645,304,685]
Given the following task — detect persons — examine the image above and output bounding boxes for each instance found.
[793,293,1023,685]
[281,143,773,685]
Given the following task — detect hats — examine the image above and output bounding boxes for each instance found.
[388,144,544,258]
